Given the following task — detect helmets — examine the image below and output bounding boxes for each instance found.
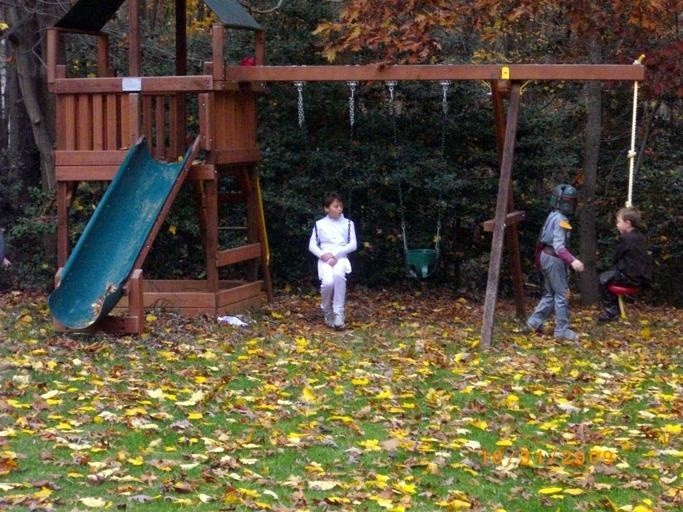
[550,184,577,214]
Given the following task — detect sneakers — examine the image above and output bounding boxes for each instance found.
[554,329,576,339]
[527,320,544,335]
[598,315,617,323]
[325,313,334,328]
[334,315,345,328]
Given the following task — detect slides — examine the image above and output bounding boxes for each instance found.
[48,135,200,331]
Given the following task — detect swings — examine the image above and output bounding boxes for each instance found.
[384,81,454,278]
[608,80,640,296]
[295,81,358,273]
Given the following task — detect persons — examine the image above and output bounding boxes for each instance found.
[308,193,358,330]
[594,208,648,324]
[527,184,584,341]
